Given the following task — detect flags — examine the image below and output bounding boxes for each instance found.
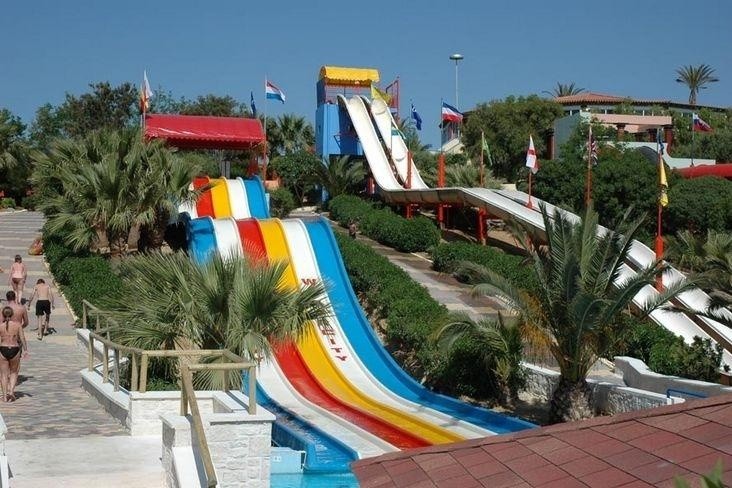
[441,102,463,123]
[391,121,407,139]
[657,153,669,208]
[265,80,286,104]
[371,85,391,104]
[482,133,492,166]
[586,129,599,166]
[525,134,538,174]
[249,91,256,118]
[138,74,154,114]
[692,113,713,132]
[411,105,422,131]
[658,128,665,155]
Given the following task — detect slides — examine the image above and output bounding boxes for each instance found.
[334,93,731,385]
[179,175,543,476]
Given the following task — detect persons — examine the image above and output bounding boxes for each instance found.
[9,254,27,305]
[0,291,28,329]
[28,279,55,340]
[0,306,29,402]
[349,222,356,239]
[28,239,43,255]
